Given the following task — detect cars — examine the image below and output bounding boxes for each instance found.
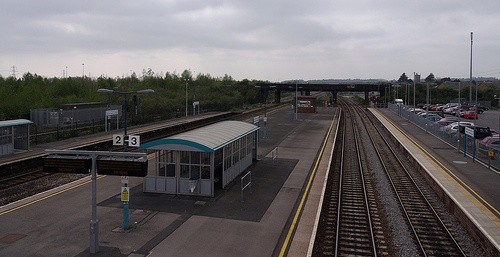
[403,102,500,154]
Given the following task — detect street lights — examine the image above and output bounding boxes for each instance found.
[41,150,149,253]
[255,85,276,117]
[289,86,303,112]
[97,88,155,136]
